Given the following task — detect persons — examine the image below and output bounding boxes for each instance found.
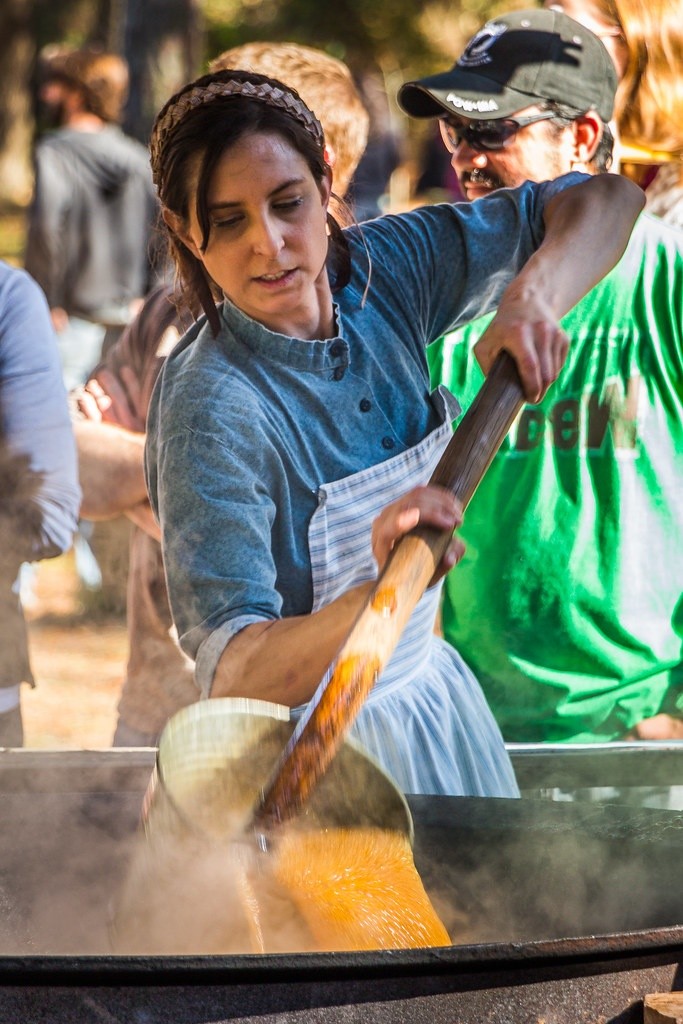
[141,66,651,801]
[0,256,84,749]
[27,0,683,748]
[394,5,683,807]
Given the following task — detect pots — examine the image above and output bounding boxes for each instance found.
[0,750,683,1024]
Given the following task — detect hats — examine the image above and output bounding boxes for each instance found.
[396,9,617,123]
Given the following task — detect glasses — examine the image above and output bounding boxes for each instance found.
[438,112,556,154]
